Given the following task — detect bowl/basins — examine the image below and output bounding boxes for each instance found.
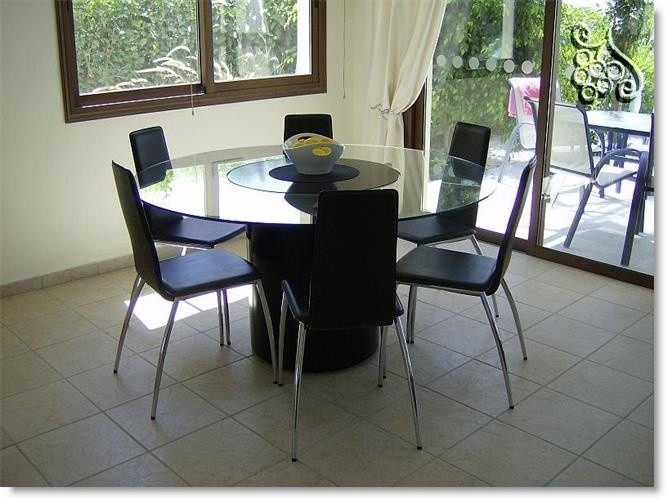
[279,131,345,175]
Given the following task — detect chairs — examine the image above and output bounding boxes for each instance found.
[381,154,538,409]
[277,190,422,462]
[524,95,645,248]
[111,159,278,421]
[620,108,654,266]
[497,77,587,213]
[128,125,248,347]
[283,113,333,145]
[394,121,499,345]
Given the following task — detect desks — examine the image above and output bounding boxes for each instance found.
[133,143,499,375]
[557,110,655,214]
